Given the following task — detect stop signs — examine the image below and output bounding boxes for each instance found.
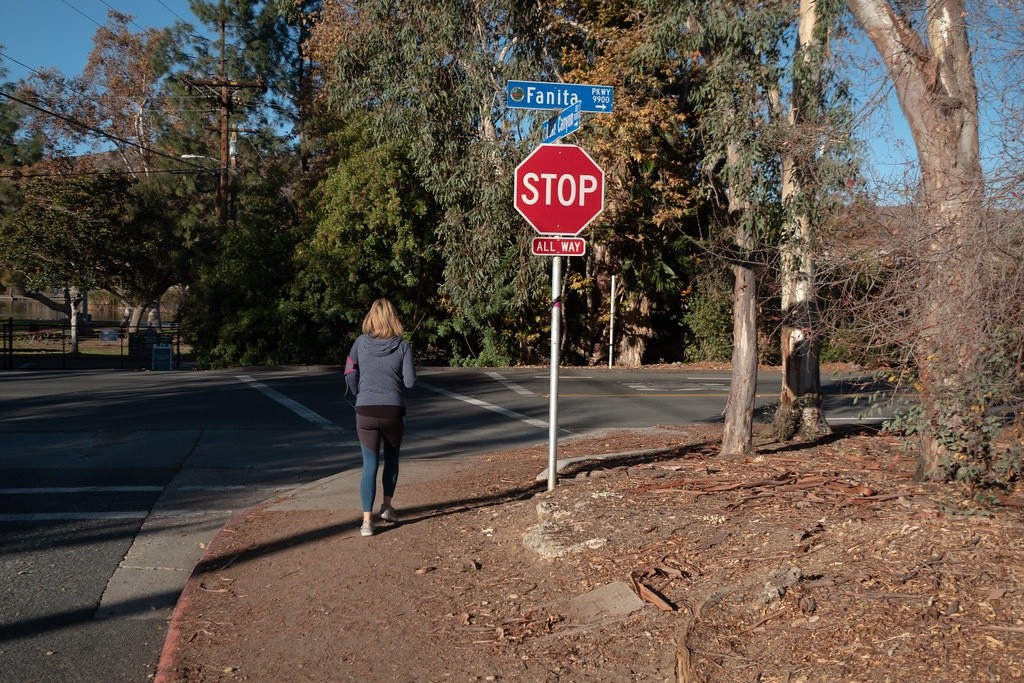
[514,143,605,236]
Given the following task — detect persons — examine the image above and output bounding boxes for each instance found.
[344,299,416,537]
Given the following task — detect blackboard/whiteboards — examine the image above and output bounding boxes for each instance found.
[153,344,173,369]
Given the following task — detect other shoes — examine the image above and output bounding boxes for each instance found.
[360,521,374,536]
[378,504,398,522]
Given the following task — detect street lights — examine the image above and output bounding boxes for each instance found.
[182,154,240,219]
